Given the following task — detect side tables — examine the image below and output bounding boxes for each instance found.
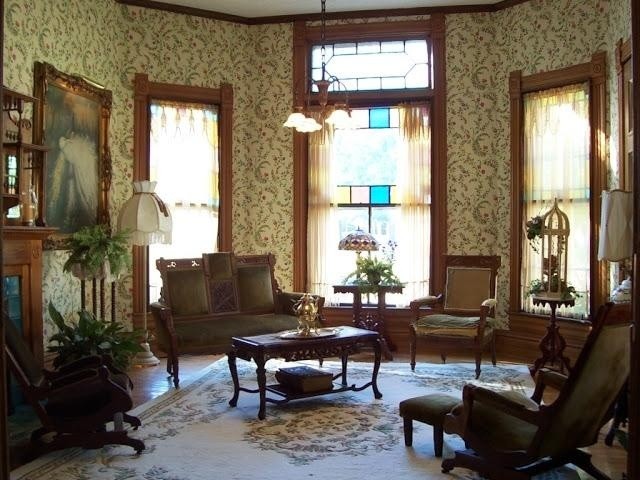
[330,283,404,362]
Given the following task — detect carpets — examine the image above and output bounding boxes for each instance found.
[7,350,590,480]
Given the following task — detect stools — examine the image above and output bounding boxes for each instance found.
[398,394,459,458]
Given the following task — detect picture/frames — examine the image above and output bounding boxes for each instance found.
[32,59,114,248]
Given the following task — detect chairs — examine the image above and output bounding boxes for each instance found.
[441,303,633,477]
[1,307,147,457]
[408,255,504,379]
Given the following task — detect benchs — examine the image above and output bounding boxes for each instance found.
[226,325,383,420]
[150,250,333,390]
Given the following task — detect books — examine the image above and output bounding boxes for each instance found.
[275,365,333,395]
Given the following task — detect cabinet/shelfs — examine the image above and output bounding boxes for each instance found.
[1,84,62,415]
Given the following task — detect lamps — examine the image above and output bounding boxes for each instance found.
[117,178,173,248]
[338,225,380,281]
[282,1,353,135]
[595,186,635,301]
